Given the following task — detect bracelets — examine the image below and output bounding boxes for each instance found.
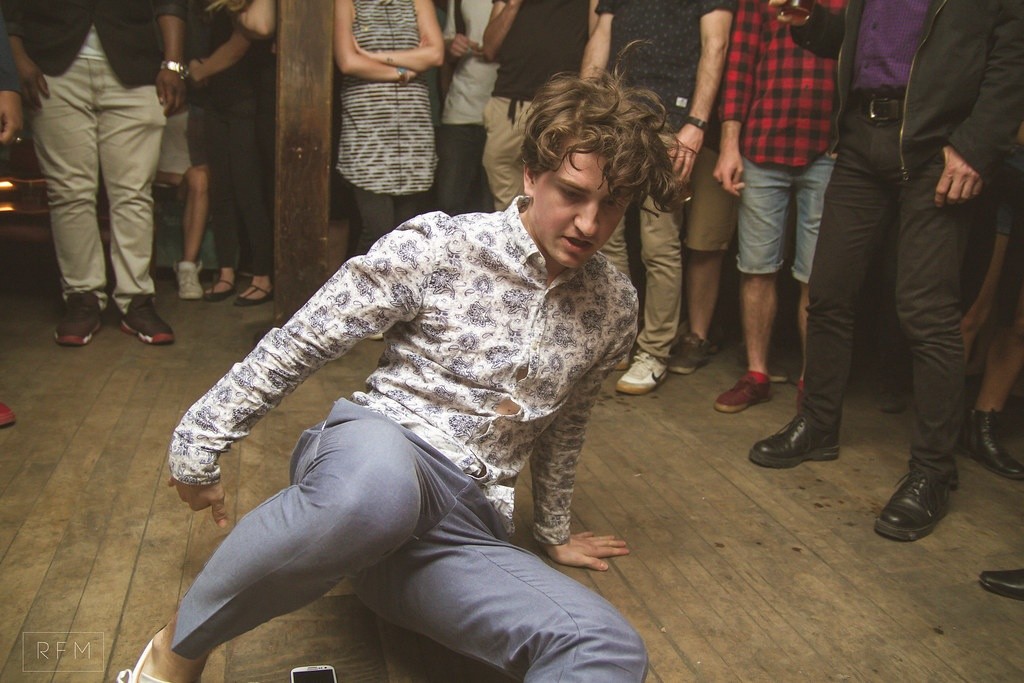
[685,115,708,131]
[398,66,409,85]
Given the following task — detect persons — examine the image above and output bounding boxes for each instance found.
[578,0,739,396]
[0,0,187,346]
[665,0,1024,541]
[184,0,274,307]
[979,568,1024,601]
[147,102,209,300]
[113,74,673,683]
[327,0,603,311]
[0,15,25,427]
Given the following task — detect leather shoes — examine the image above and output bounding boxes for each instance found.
[875,462,959,541]
[979,568,1024,601]
[749,415,841,469]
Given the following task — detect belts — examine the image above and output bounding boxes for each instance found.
[848,92,904,122]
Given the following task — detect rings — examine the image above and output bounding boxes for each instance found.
[12,137,22,144]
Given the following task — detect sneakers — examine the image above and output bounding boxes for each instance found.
[172,260,206,300]
[120,294,175,345]
[615,349,668,395]
[52,291,100,344]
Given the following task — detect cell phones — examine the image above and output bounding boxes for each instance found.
[291,665,337,683]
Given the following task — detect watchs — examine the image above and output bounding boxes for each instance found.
[161,60,189,79]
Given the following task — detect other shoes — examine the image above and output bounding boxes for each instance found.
[204,280,235,301]
[715,376,770,412]
[669,333,708,373]
[236,283,274,307]
[875,387,907,413]
[116,636,203,683]
[768,343,788,382]
[0,401,15,427]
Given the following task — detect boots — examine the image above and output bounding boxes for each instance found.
[958,407,1024,480]
